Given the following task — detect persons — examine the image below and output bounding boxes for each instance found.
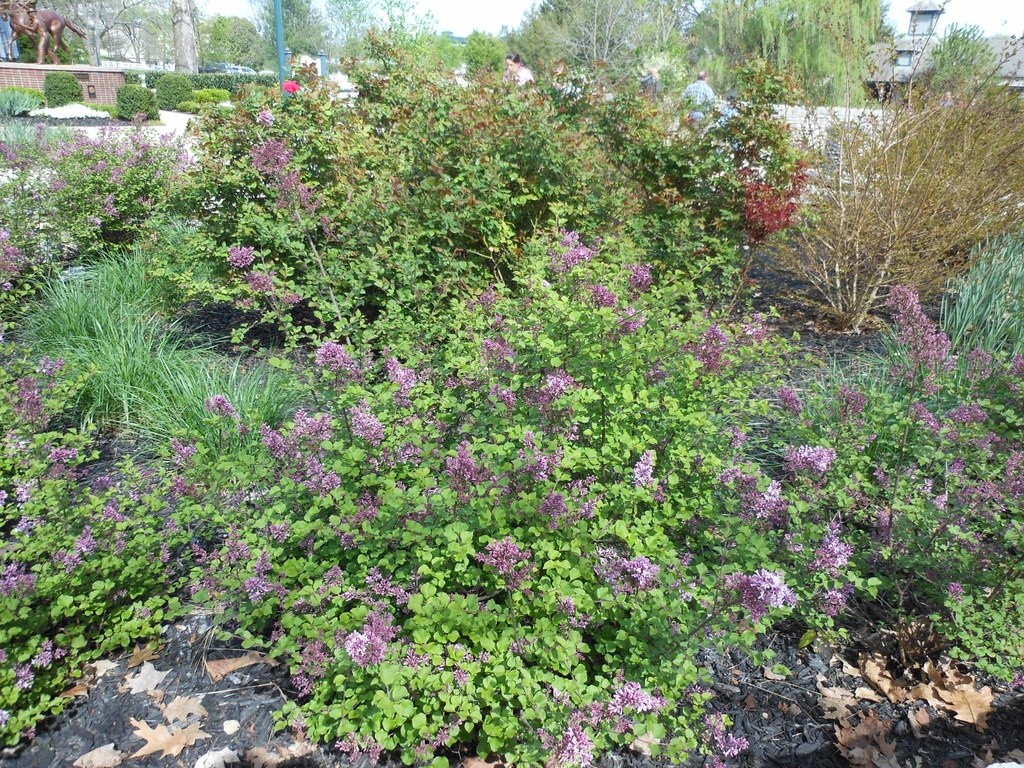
[499,50,538,90]
[678,70,720,120]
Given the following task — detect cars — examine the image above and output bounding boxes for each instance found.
[232,67,256,74]
[198,62,230,74]
